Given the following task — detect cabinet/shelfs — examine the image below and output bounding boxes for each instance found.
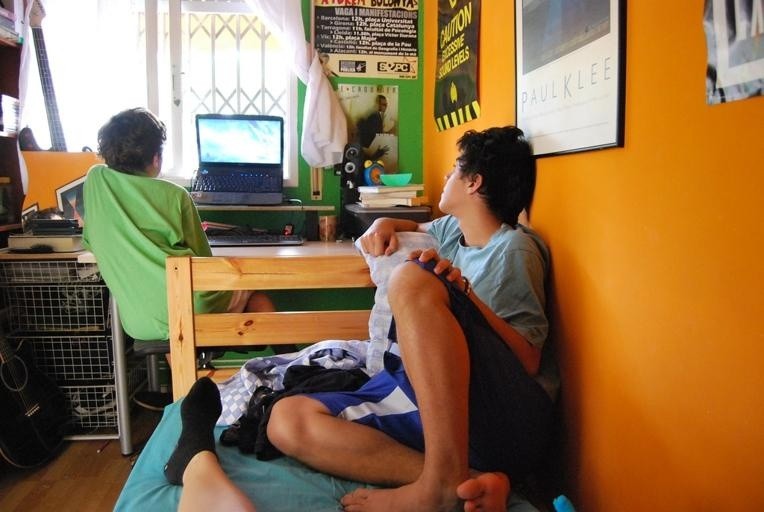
[0,247,146,441]
[0,0,32,232]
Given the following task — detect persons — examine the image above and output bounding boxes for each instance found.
[264,125,552,512]
[80,106,298,375]
[357,92,389,147]
[370,144,391,160]
[161,376,257,512]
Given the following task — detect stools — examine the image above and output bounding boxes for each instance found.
[128,337,269,413]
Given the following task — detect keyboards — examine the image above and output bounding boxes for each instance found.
[207,236,303,246]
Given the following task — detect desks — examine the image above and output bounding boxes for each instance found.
[78,238,361,457]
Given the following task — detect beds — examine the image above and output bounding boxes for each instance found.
[111,251,574,512]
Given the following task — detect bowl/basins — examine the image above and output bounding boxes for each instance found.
[380,173,412,186]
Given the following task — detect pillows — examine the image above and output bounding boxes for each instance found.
[353,232,442,377]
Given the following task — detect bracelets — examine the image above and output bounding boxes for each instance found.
[460,273,473,299]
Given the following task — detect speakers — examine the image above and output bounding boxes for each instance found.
[340,144,364,187]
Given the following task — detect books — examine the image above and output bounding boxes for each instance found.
[0,7,20,42]
[7,233,84,252]
[0,93,19,133]
[355,183,430,209]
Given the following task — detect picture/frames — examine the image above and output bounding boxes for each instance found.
[512,1,627,159]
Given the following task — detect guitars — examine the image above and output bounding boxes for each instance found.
[0,331,73,470]
[19,0,104,232]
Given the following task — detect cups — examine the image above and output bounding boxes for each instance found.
[319,215,337,242]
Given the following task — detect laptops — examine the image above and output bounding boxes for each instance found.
[191,114,283,205]
